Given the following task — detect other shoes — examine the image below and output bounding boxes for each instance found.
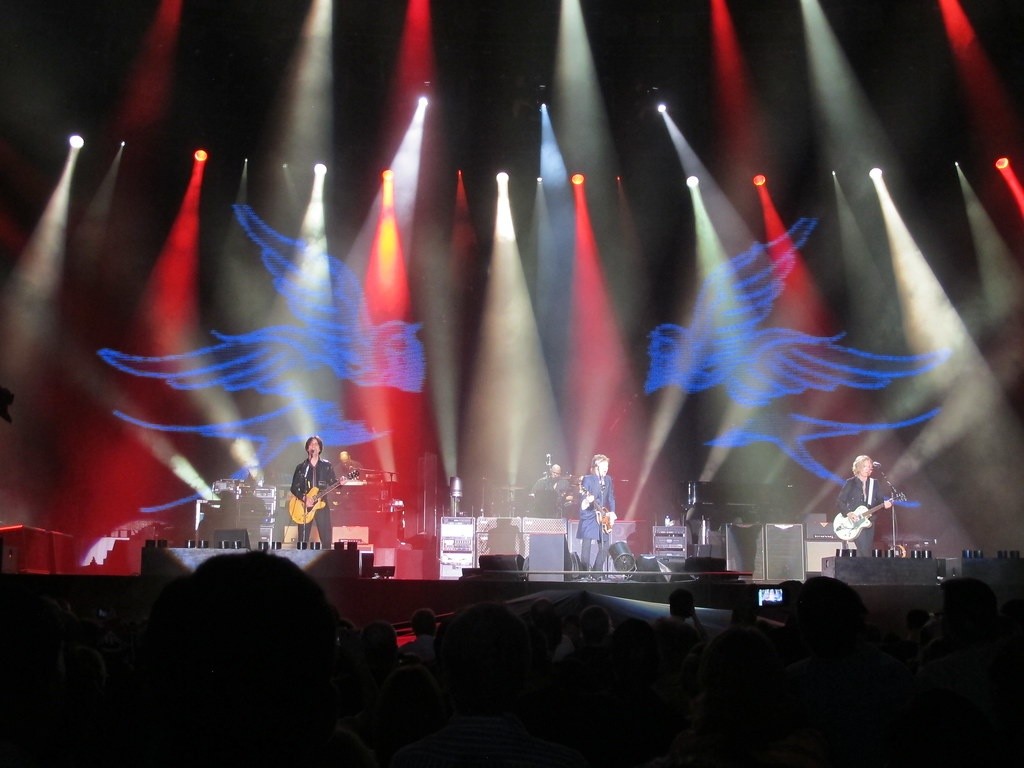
[577,576,612,582]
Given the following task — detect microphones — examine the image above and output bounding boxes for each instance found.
[594,461,601,467]
[546,454,551,466]
[872,462,881,467]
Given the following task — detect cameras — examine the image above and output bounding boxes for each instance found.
[756,584,784,608]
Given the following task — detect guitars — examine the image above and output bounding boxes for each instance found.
[580,485,612,535]
[288,469,361,525]
[833,492,907,542]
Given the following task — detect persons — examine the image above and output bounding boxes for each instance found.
[290,436,346,549]
[576,454,617,579]
[835,455,895,557]
[532,464,573,518]
[0,553,1024,768]
[236,479,268,536]
[334,451,364,479]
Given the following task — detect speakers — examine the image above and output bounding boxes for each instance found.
[723,523,766,581]
[214,529,250,548]
[0,525,78,576]
[477,517,726,582]
[767,523,962,586]
